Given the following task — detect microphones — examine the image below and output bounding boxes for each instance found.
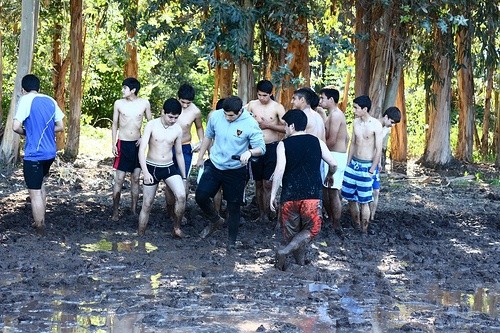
[232,155,258,162]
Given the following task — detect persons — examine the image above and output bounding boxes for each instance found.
[341,95,385,239]
[137,97,187,239]
[269,109,338,271]
[13,74,67,228]
[162,80,402,224]
[193,95,267,256]
[109,77,153,224]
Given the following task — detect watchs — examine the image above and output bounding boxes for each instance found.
[247,150,253,157]
